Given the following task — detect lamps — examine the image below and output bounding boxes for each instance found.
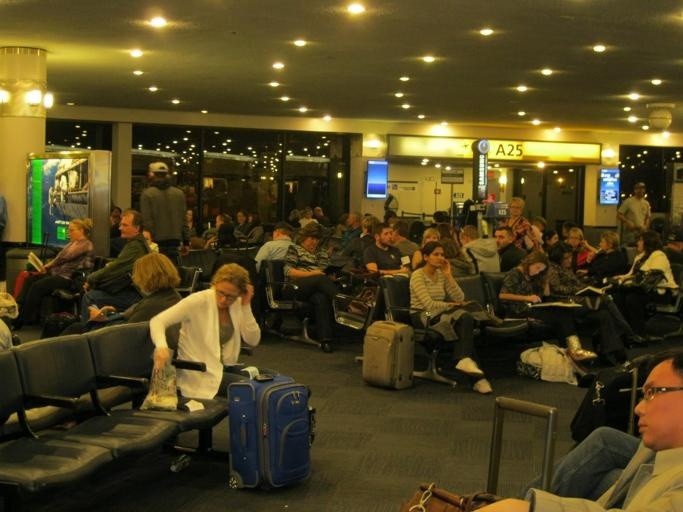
[644,100,676,131]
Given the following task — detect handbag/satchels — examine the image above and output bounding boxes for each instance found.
[346,286,376,316]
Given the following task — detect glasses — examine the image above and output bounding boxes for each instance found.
[645,385,682,399]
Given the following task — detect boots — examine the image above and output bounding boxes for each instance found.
[565,335,598,361]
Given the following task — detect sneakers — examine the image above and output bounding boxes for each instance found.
[455,357,484,376]
[473,379,493,394]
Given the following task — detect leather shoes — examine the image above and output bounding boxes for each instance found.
[321,341,334,353]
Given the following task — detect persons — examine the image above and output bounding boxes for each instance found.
[473,349,683,512]
[150,262,279,399]
[515,425,642,508]
[617,181,651,245]
[184,198,683,392]
[139,161,190,261]
[0,199,181,354]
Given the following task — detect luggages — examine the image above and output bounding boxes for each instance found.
[362,321,415,388]
[569,367,634,444]
[227,375,316,490]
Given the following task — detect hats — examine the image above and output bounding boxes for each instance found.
[148,162,169,173]
